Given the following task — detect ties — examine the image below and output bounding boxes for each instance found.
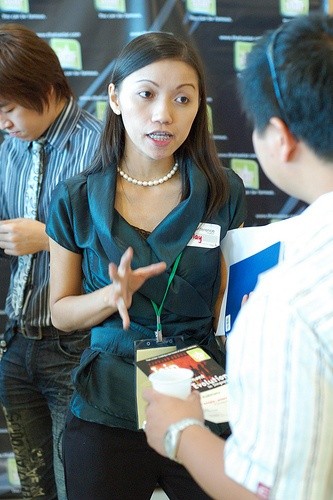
[11,137,48,316]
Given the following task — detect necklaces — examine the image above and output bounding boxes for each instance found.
[116,163,178,187]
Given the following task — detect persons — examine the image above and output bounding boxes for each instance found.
[0,24,107,500]
[142,11,333,500]
[45,32,249,500]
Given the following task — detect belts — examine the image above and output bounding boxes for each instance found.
[16,323,60,340]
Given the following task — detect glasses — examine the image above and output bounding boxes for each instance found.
[265,23,299,141]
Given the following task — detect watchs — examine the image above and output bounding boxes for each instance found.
[163,417,208,461]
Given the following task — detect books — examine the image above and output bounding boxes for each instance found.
[224,241,283,335]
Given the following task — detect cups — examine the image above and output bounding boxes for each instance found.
[148,367,195,401]
